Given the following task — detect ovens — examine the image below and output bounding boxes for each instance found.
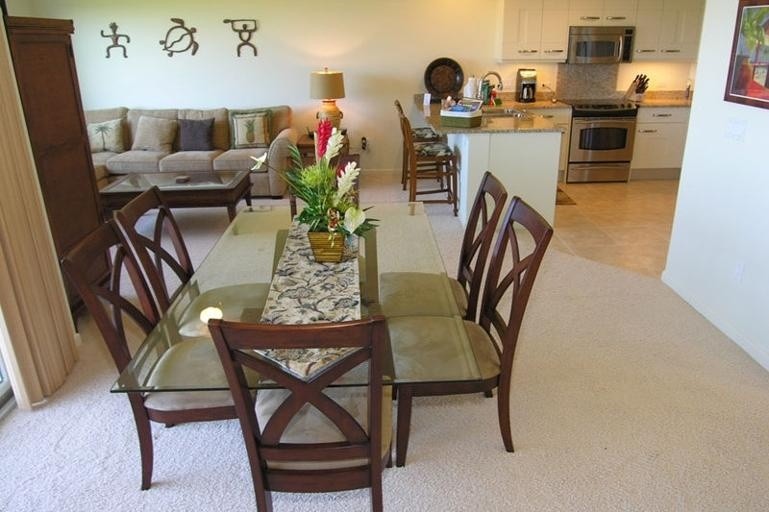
[566,113,635,184]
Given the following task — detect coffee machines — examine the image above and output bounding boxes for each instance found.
[515,68,538,102]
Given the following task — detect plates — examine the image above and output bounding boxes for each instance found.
[423,57,464,98]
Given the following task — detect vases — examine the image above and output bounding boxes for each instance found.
[304,232,345,262]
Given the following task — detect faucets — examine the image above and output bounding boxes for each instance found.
[542,83,557,101]
[478,71,503,100]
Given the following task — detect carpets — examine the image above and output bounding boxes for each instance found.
[556,183,577,205]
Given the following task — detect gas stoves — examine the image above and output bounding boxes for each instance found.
[561,97,639,114]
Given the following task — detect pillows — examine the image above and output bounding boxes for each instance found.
[130,115,172,149]
[176,118,213,149]
[229,112,273,149]
[85,116,126,155]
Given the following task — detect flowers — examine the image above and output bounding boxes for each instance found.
[250,123,381,240]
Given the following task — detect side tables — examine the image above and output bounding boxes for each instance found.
[295,134,359,206]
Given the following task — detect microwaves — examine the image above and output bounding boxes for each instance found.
[568,24,636,63]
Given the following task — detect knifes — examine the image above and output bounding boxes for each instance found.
[632,73,649,95]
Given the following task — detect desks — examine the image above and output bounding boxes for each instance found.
[111,204,479,402]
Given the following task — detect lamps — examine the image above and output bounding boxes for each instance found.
[308,65,348,138]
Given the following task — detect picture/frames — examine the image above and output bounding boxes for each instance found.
[722,0,769,110]
[456,98,485,113]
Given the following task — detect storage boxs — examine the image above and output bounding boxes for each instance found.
[440,108,483,129]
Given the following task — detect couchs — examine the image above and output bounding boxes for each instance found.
[85,106,295,199]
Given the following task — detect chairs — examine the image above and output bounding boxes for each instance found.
[391,97,448,193]
[206,313,386,510]
[376,168,510,399]
[112,183,271,340]
[377,194,553,464]
[398,116,459,216]
[58,216,258,493]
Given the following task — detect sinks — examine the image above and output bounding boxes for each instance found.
[483,108,528,117]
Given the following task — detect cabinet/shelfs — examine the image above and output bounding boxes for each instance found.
[531,107,573,170]
[628,107,691,171]
[490,0,569,63]
[2,14,115,331]
[570,0,637,26]
[632,0,703,61]
